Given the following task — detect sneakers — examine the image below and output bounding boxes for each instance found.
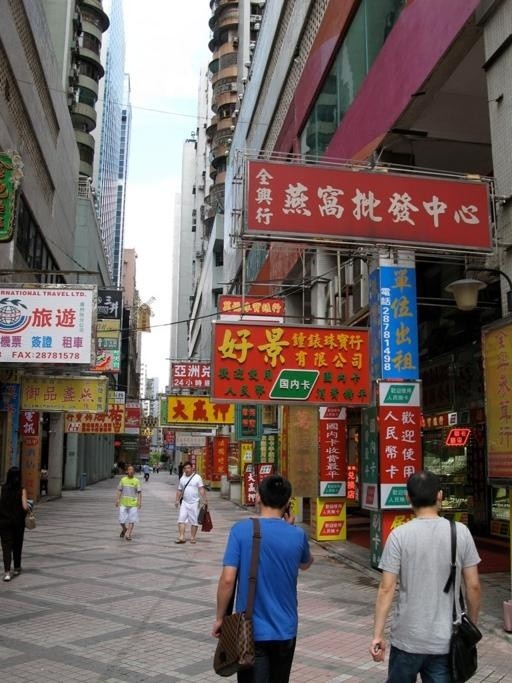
[4,567,21,581]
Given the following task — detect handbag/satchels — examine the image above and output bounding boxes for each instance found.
[24,511,37,530]
[201,511,213,533]
[198,504,205,524]
[179,496,183,505]
[448,612,483,683]
[212,609,258,679]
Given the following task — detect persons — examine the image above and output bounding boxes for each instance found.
[115,466,142,540]
[1,466,35,581]
[174,461,213,544]
[178,461,184,480]
[144,463,150,478]
[211,474,314,681]
[368,469,485,682]
[168,462,174,474]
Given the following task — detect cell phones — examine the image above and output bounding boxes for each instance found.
[282,502,291,518]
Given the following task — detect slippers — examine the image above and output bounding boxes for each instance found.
[174,537,196,544]
[119,528,133,540]
